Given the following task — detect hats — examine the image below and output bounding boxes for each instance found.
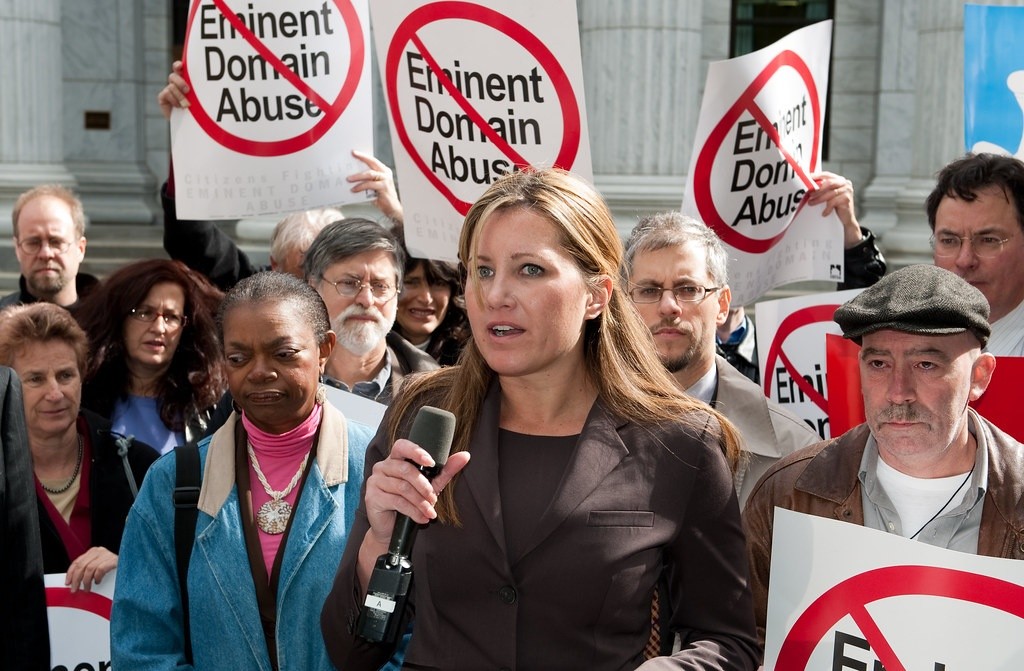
[833,263,994,351]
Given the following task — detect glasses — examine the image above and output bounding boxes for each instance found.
[627,284,719,304]
[15,235,77,256]
[322,275,401,300]
[128,307,188,329]
[927,231,1011,259]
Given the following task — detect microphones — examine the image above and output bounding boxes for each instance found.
[358,405,458,643]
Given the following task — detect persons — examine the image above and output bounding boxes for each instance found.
[76,259,226,456]
[158,61,405,293]
[716,171,887,387]
[110,271,413,671]
[319,167,761,671]
[741,264,1024,671]
[0,183,103,315]
[0,302,162,593]
[924,152,1024,357]
[385,224,472,373]
[620,209,824,515]
[304,218,442,407]
